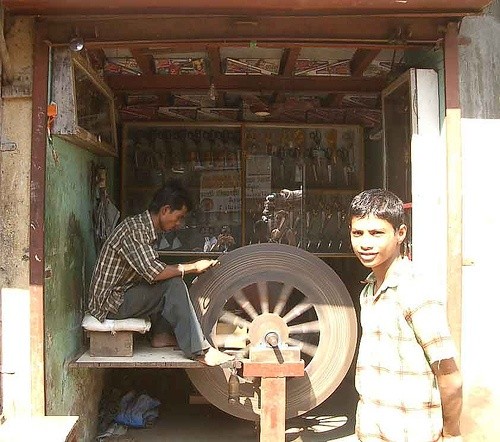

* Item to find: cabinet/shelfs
[120,123,365,263]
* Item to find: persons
[249,210,296,247]
[348,189,463,442]
[203,226,236,252]
[89,186,236,366]
